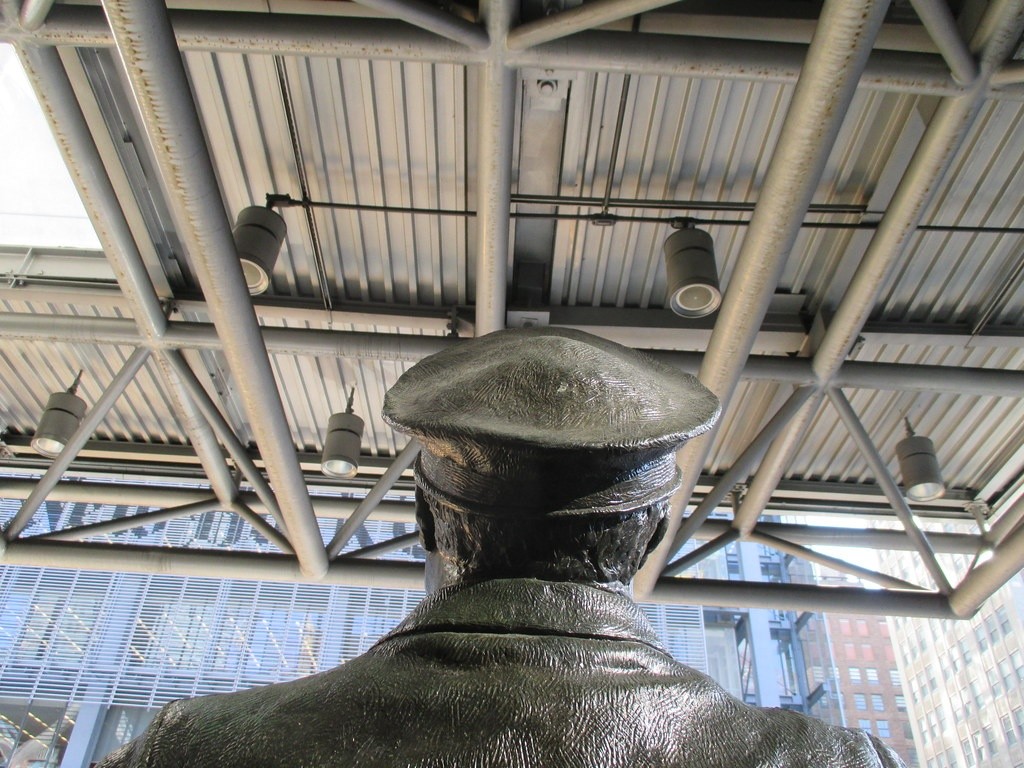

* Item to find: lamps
[895,416,947,502]
[230,193,291,297]
[321,386,364,478]
[31,368,87,458]
[664,221,722,318]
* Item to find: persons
[89,325,912,768]
